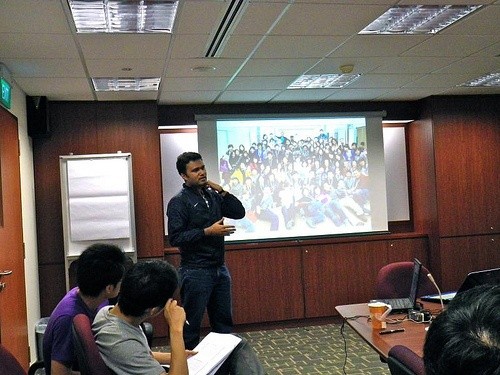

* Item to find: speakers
[27,96,50,137]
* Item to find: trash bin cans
[36,317,52,362]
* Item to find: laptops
[370,258,421,311]
[421,268,500,304]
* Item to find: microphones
[421,267,445,311]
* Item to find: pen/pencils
[170,299,190,324]
[378,328,405,334]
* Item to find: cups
[368,303,391,329]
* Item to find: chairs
[70,313,114,375]
[375,261,431,298]
[387,345,425,375]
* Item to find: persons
[91,259,268,375]
[220,129,370,232]
[423,284,500,375]
[166,152,245,350]
[43,244,126,375]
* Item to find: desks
[333,299,445,364]
